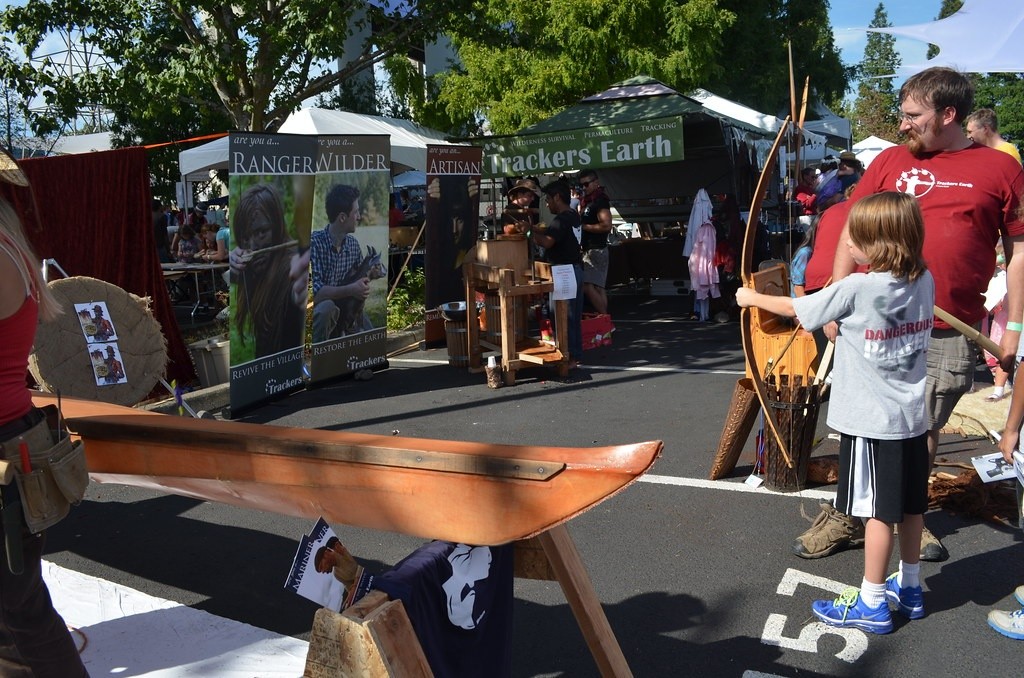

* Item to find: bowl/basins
[438,302,485,321]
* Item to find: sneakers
[918,525,947,560]
[791,498,866,558]
[885,571,925,619]
[1015,585,1024,606]
[987,609,1024,640]
[812,588,894,634]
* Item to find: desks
[161,261,228,326]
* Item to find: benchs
[746,263,831,385]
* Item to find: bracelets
[207,255,210,259]
[1005,322,1024,331]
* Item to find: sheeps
[329,246,388,338]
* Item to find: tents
[472,75,851,196]
[182,107,472,226]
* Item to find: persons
[229,184,310,359]
[791,66,1024,639]
[104,346,125,384]
[578,168,616,332]
[736,191,936,634]
[310,185,388,343]
[0,197,91,678]
[326,536,352,558]
[531,180,583,265]
[92,305,114,341]
[502,179,541,258]
[314,546,363,610]
[152,199,229,307]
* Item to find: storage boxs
[541,313,612,354]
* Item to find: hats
[0,145,28,187]
[837,153,855,160]
[508,179,543,197]
[196,202,208,215]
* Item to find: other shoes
[189,308,199,317]
[576,360,583,368]
[985,394,1004,402]
[610,323,616,333]
[197,306,210,315]
[567,361,576,370]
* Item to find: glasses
[579,179,594,188]
[899,105,945,125]
[810,175,817,179]
[545,197,553,203]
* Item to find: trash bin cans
[189,332,230,389]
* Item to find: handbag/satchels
[0,410,89,535]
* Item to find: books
[283,515,373,614]
